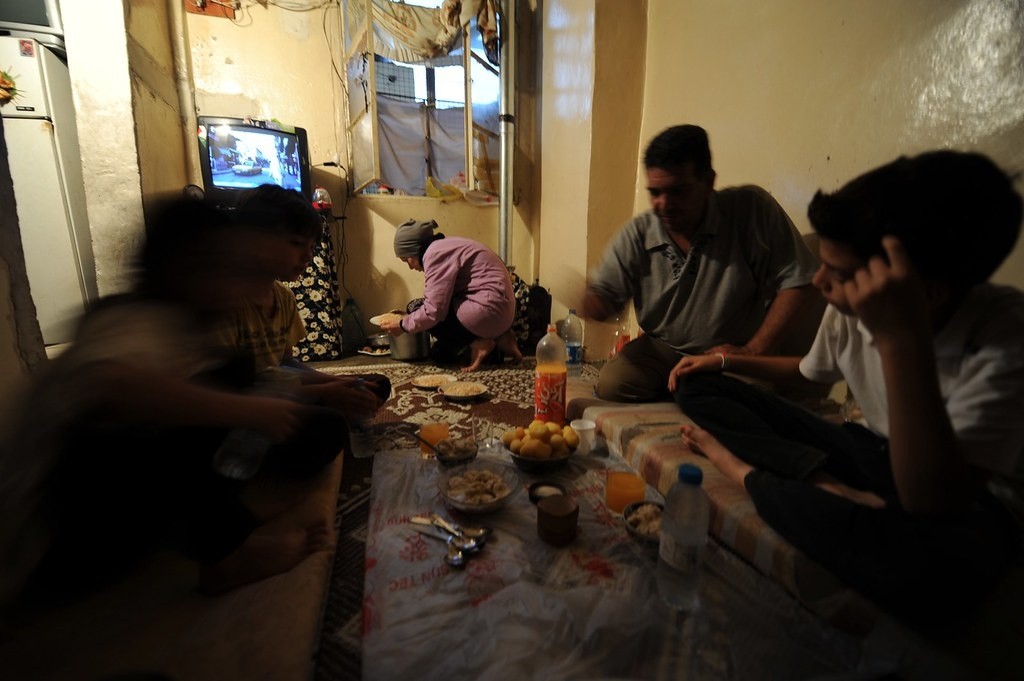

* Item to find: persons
[662,149,1023,657]
[551,119,813,407]
[377,217,523,374]
[0,182,333,676]
[200,181,395,478]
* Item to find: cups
[537,494,579,549]
[471,401,494,453]
[605,464,647,520]
[571,420,596,458]
[419,421,451,460]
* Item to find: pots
[389,327,430,362]
[366,334,389,346]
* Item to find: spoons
[409,511,493,568]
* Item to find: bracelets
[400,317,412,334]
[713,351,731,370]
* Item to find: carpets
[313,363,658,680]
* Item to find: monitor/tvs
[198,115,311,203]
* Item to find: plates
[411,374,458,388]
[358,349,391,355]
[437,380,487,398]
[369,315,383,325]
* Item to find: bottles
[657,464,710,610]
[559,310,583,386]
[343,378,376,459]
[534,324,566,429]
[608,307,631,364]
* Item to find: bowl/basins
[622,501,668,550]
[434,440,479,468]
[502,442,578,473]
[436,460,520,513]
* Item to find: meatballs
[504,420,580,460]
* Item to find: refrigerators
[0,36,101,346]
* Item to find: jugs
[312,188,333,209]
